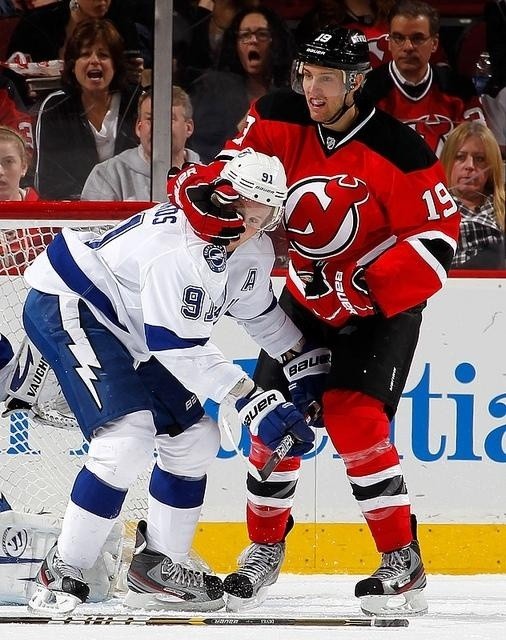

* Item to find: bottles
[471,51,492,93]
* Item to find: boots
[224,514,293,598]
[35,540,90,603]
[126,521,223,602]
[354,514,426,599]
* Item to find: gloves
[282,346,334,427]
[235,388,315,457]
[303,260,378,328]
[167,158,246,246]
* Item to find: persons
[167,26,461,599]
[0,0,506,268]
[22,146,332,605]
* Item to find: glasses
[236,29,274,44]
[386,31,435,47]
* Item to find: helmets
[221,147,290,233]
[290,27,373,98]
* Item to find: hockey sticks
[221,399,321,482]
